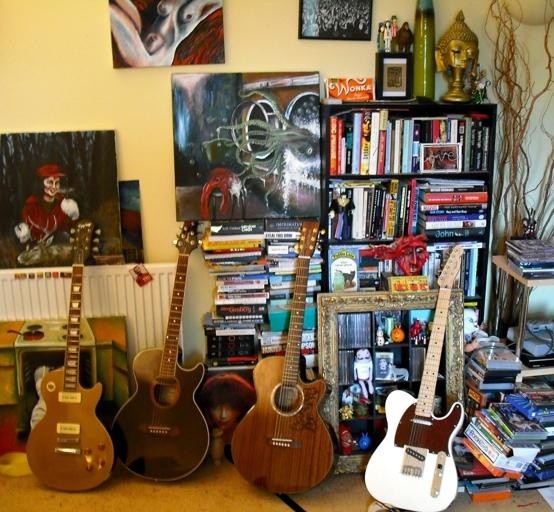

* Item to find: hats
[35,162,66,178]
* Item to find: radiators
[0,263,185,395]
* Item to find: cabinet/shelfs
[320,101,498,326]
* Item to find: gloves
[14,222,31,244]
[61,197,80,221]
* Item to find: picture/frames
[376,52,413,100]
[299,0,372,40]
[317,289,465,476]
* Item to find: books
[329,177,488,241]
[329,111,490,174]
[452,343,554,503]
[505,239,554,279]
[201,220,324,367]
[329,243,483,386]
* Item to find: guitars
[26,220,115,492]
[365,243,466,512]
[108,219,211,483]
[231,218,340,494]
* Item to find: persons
[435,10,480,103]
[383,21,391,54]
[202,373,257,466]
[338,349,374,455]
[131,266,151,286]
[317,1,370,39]
[376,22,386,53]
[13,165,79,251]
[390,15,398,54]
